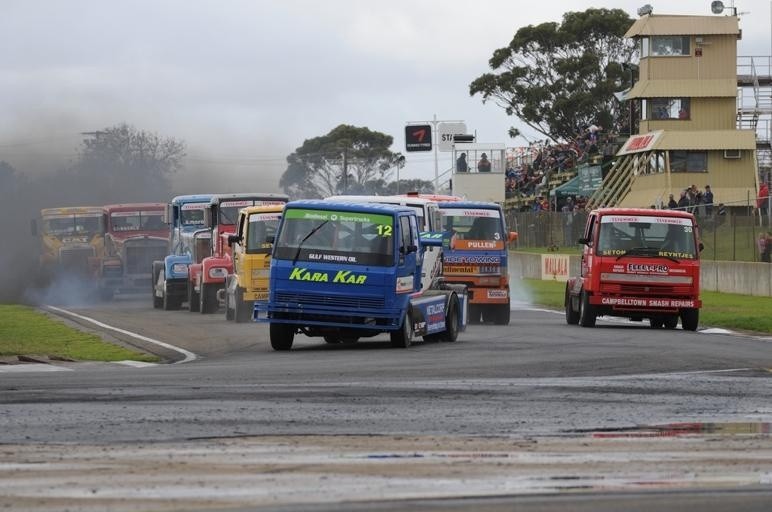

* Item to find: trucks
[187,193,289,314]
[434,201,518,325]
[151,195,215,311]
[322,195,469,332]
[28,206,104,292]
[252,199,461,351]
[384,193,462,203]
[225,204,288,324]
[564,207,704,330]
[89,203,171,302]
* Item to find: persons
[758,231,772,263]
[457,153,467,172]
[504,106,726,247]
[296,221,331,247]
[757,180,769,226]
[478,153,490,172]
